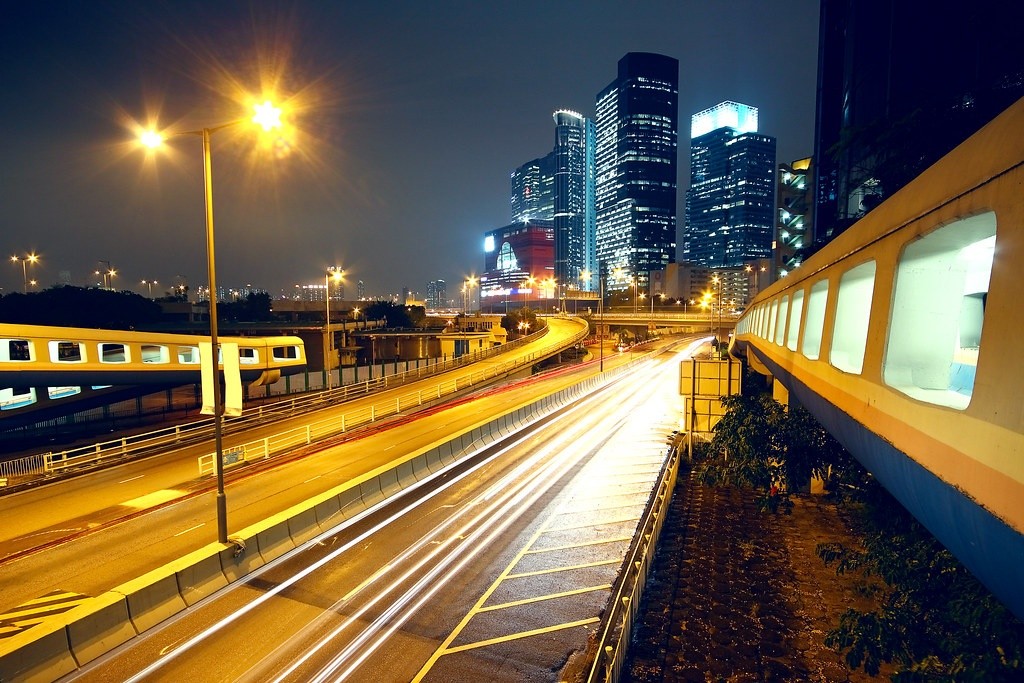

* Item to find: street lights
[525,278,534,335]
[519,279,555,323]
[142,105,277,543]
[630,276,644,317]
[640,294,665,352]
[746,266,765,296]
[706,294,713,336]
[464,280,475,354]
[714,278,721,361]
[9,255,186,297]
[581,271,622,372]
[677,301,694,319]
[358,291,456,315]
[326,272,342,400]
